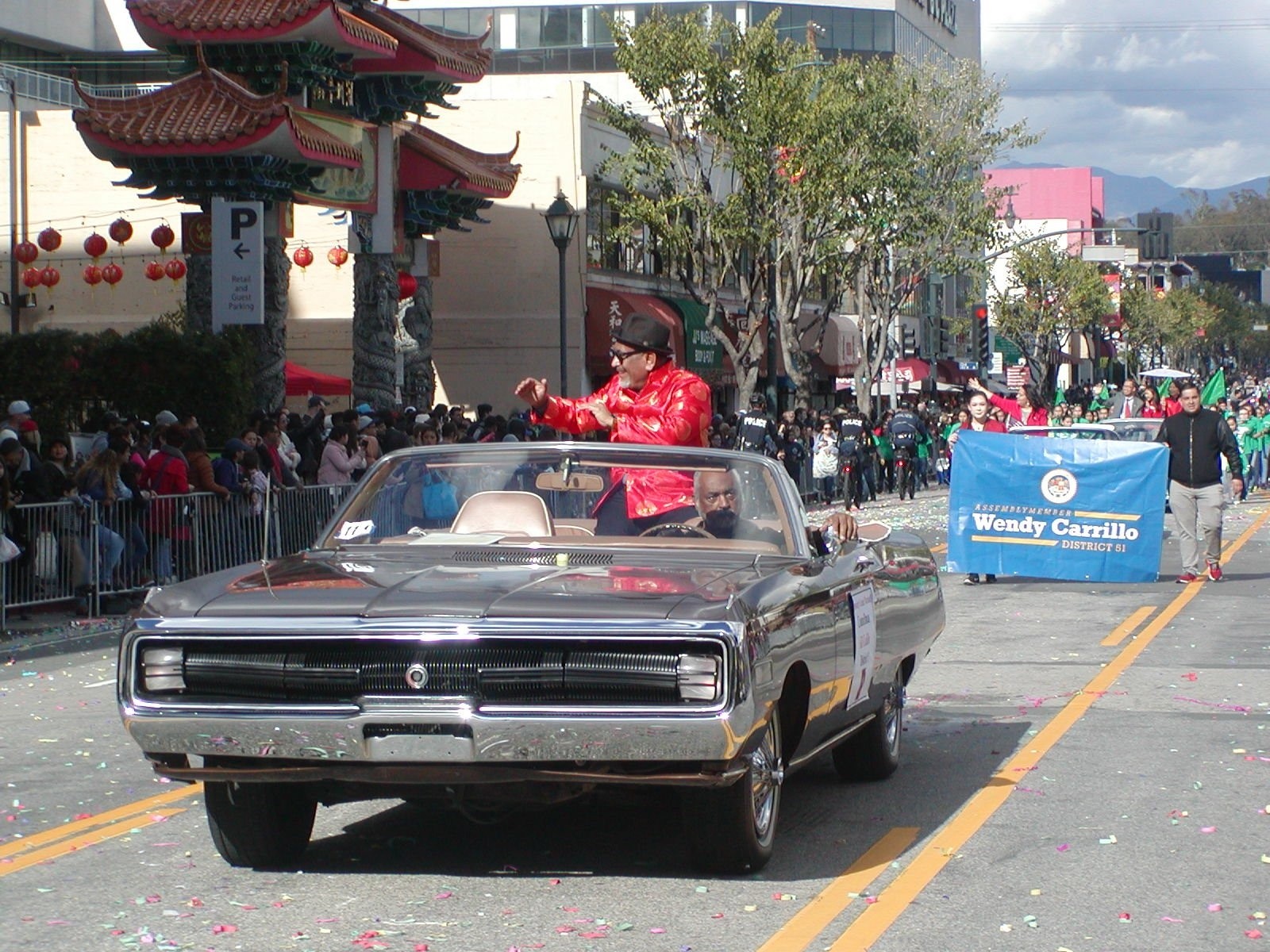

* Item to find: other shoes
[99,582,118,592]
[1175,571,1196,584]
[963,573,980,585]
[75,584,95,594]
[133,576,155,586]
[1205,559,1222,581]
[76,605,94,617]
[986,573,997,583]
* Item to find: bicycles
[892,445,915,500]
[833,437,880,510]
[728,448,784,520]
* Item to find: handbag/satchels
[0,534,21,563]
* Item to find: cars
[115,440,945,873]
[1002,417,1171,511]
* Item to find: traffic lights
[934,314,950,354]
[975,305,988,321]
[900,323,915,360]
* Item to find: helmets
[847,405,860,412]
[748,392,765,403]
[900,400,910,407]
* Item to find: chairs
[448,490,556,540]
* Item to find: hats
[96,409,121,431]
[155,410,178,426]
[225,438,250,457]
[307,396,330,408]
[7,400,30,415]
[610,312,675,357]
[357,416,373,432]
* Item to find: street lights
[541,191,582,442]
[765,61,836,452]
[940,192,1020,359]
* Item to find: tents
[285,360,351,409]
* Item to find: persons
[968,377,1048,436]
[1202,374,1270,504]
[682,468,858,548]
[710,393,969,513]
[515,313,712,535]
[1046,377,1182,440]
[946,391,1009,585]
[0,400,303,620]
[1155,383,1244,583]
[276,396,609,552]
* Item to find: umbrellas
[1139,369,1192,377]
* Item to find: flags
[1090,382,1109,411]
[1158,377,1173,397]
[1201,370,1226,405]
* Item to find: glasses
[608,346,647,365]
[280,418,290,425]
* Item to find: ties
[1125,399,1130,418]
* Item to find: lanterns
[15,218,187,302]
[396,269,417,302]
[293,246,314,272]
[328,246,348,269]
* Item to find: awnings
[584,285,1041,394]
[1094,339,1117,358]
[1049,348,1081,364]
